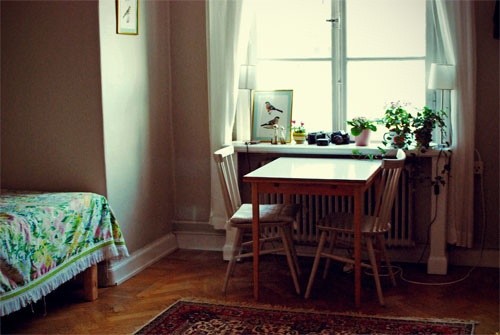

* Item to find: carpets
[131,296,477,335]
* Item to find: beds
[0,189,129,322]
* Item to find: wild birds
[260,116,280,129]
[265,102,283,115]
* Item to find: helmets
[264,97,276,107]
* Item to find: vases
[292,132,306,145]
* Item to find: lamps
[427,61,457,147]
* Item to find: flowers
[293,120,306,131]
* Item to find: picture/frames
[115,0,140,35]
[249,88,292,144]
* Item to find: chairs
[213,145,302,295]
[305,148,406,307]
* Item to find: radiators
[258,159,417,249]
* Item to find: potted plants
[347,103,446,147]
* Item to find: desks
[242,156,385,307]
